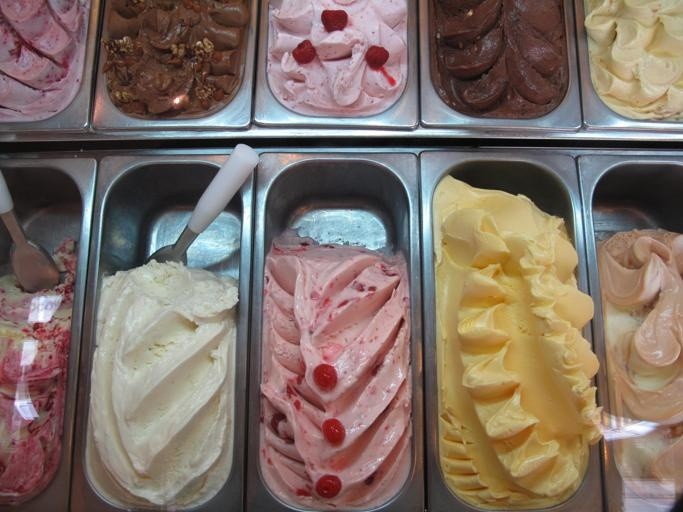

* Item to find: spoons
[1,170,60,293]
[146,143,260,267]
